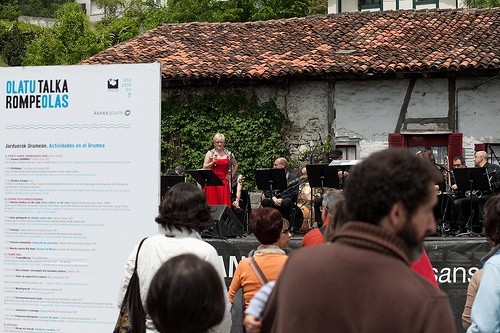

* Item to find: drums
[299,183,317,201]
[292,203,315,233]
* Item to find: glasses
[453,163,463,167]
[281,227,291,234]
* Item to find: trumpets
[443,157,454,195]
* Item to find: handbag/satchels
[113,237,148,333]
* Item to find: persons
[227,208,288,333]
[302,190,344,247]
[202,133,237,208]
[260,148,456,333]
[244,280,276,333]
[147,253,225,333]
[412,247,439,290]
[461,269,481,329]
[464,195,500,333]
[264,157,299,238]
[277,219,293,255]
[328,200,348,233]
[118,182,233,333]
[328,149,349,186]
[416,151,500,237]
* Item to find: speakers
[200,205,244,237]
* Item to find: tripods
[452,167,492,238]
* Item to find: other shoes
[469,231,480,236]
[455,228,467,235]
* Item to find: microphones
[214,153,218,167]
[450,171,453,174]
[319,134,323,145]
